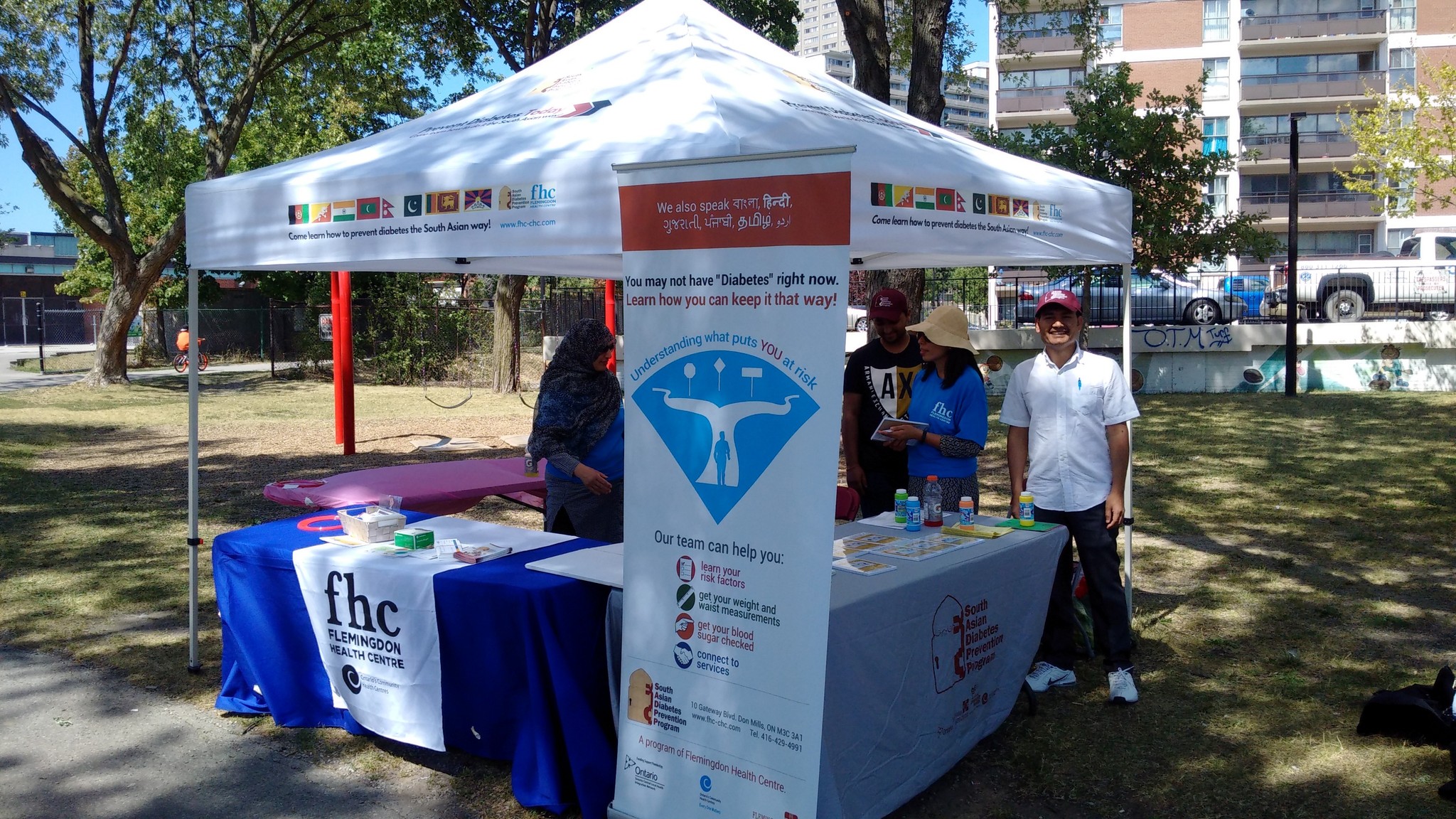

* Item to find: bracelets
[919,430,927,443]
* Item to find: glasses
[599,342,615,354]
[916,332,933,344]
[873,317,900,327]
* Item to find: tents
[181,0,1136,675]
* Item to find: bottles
[894,489,908,523]
[959,497,974,531]
[525,445,539,477]
[1019,492,1034,527]
[906,496,921,531]
[923,475,943,527]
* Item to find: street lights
[1283,110,1308,397]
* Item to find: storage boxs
[393,528,434,550]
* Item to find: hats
[1036,289,1083,317]
[905,306,980,355]
[869,288,907,321]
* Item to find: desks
[212,457,1068,818]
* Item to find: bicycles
[173,338,209,373]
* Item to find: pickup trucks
[1259,232,1456,323]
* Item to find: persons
[841,288,925,521]
[177,326,205,366]
[998,288,1140,706]
[527,317,623,543]
[878,306,988,515]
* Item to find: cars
[1216,275,1276,317]
[846,305,868,332]
[1014,263,1248,326]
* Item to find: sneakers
[1022,659,1077,691]
[1106,665,1138,703]
